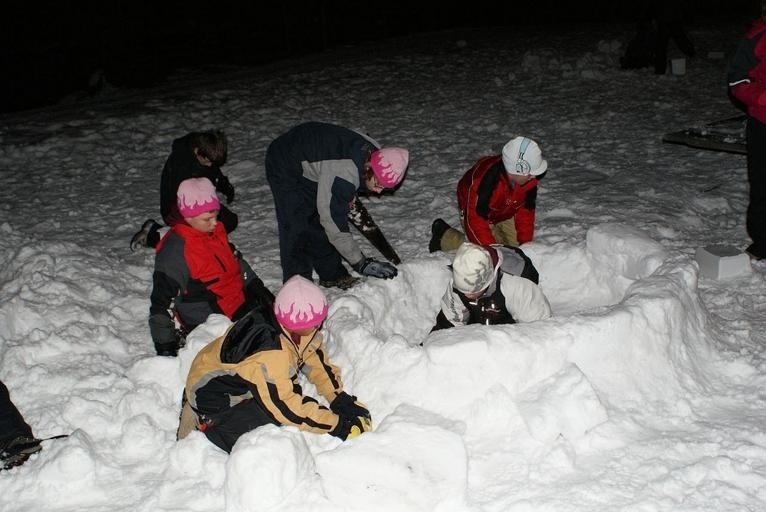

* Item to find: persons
[176,274,372,455]
[420,238,553,348]
[0,381,43,471]
[128,126,239,250]
[726,14,766,262]
[148,177,276,358]
[263,120,411,291]
[424,135,551,253]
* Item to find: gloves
[327,416,373,441]
[152,340,179,356]
[352,258,399,280]
[330,391,371,420]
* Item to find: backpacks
[487,242,538,285]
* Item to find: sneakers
[131,218,155,251]
[319,273,362,290]
[0,434,42,471]
[429,217,450,254]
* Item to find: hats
[370,144,410,190]
[450,241,502,294]
[502,136,548,176]
[274,273,329,331]
[176,177,221,218]
